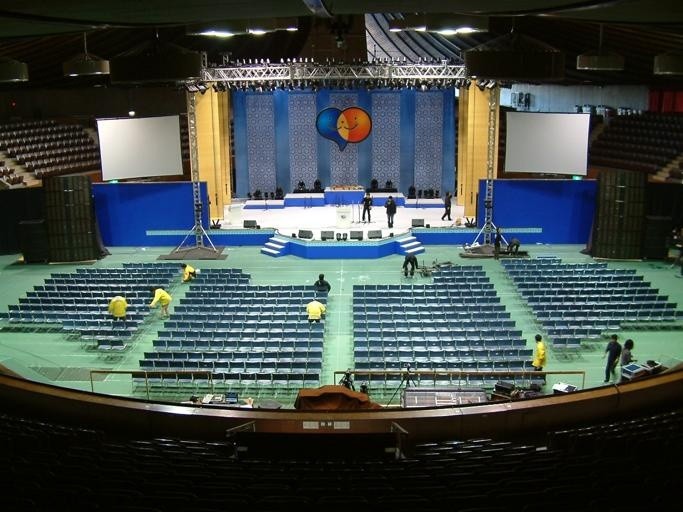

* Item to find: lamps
[2,58,28,84]
[63,32,110,79]
[575,23,681,78]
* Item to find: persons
[531,334,547,386]
[601,334,622,383]
[507,238,521,257]
[440,191,453,222]
[384,196,397,229]
[305,299,326,323]
[148,287,172,320]
[108,294,128,322]
[314,273,331,293]
[402,254,419,279]
[180,263,197,282]
[493,227,503,261]
[620,338,639,382]
[360,193,373,223]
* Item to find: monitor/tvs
[224,392,238,404]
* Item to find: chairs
[589,113,681,174]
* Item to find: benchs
[0,112,188,189]
